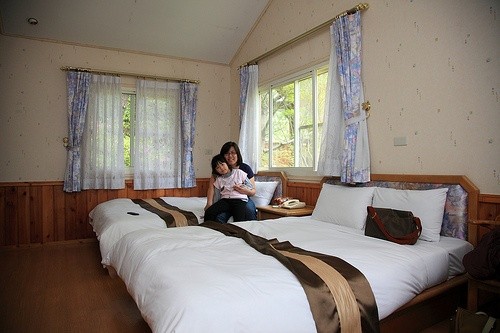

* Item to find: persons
[204,154,255,222]
[204,141,257,222]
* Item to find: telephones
[282,199,306,209]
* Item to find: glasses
[224,152,237,156]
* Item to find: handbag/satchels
[365,206,422,245]
